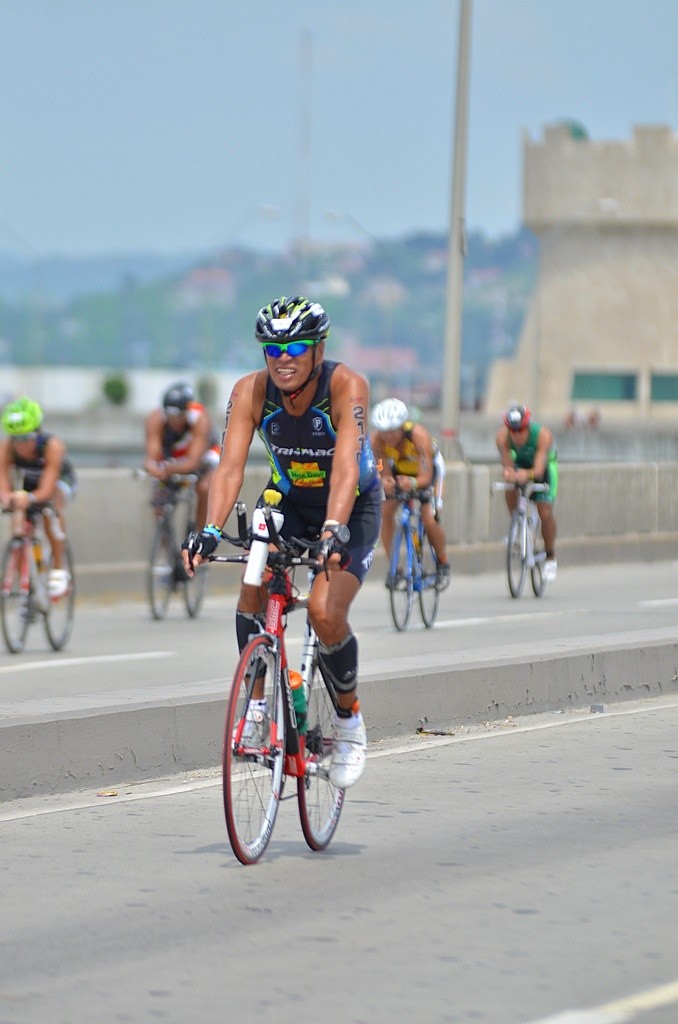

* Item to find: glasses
[259,338,320,358]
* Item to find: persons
[143,382,223,587]
[368,398,452,592]
[181,296,386,789]
[495,405,559,581]
[0,396,76,602]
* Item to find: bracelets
[203,523,223,542]
[412,478,417,490]
[531,471,534,480]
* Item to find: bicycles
[181,501,354,861]
[381,484,454,630]
[145,464,217,621]
[1,500,79,656]
[489,479,560,598]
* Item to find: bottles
[412,527,420,557]
[33,537,44,575]
[288,670,310,734]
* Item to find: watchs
[324,524,350,544]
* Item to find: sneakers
[385,568,404,588]
[230,709,272,750]
[543,555,558,581]
[327,716,366,788]
[49,566,71,599]
[433,560,450,591]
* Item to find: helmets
[372,396,409,433]
[503,404,532,432]
[2,395,42,436]
[163,383,194,411]
[257,295,330,343]
